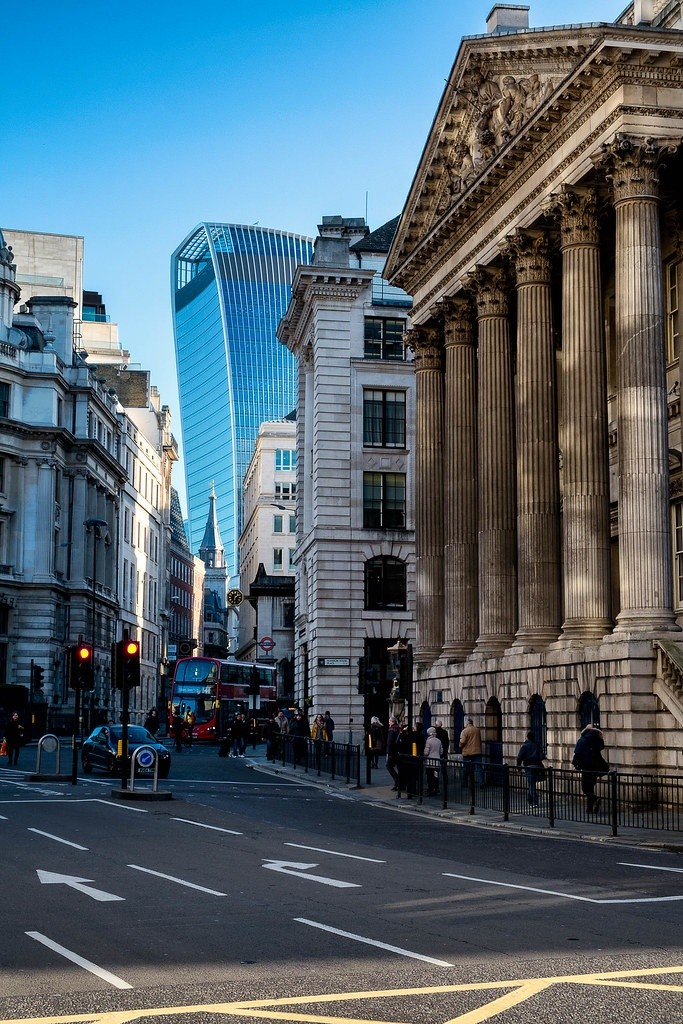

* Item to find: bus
[168,656,276,744]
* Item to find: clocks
[227,589,244,606]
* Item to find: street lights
[385,640,407,730]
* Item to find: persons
[517,732,539,809]
[2,711,25,765]
[173,711,184,753]
[434,719,449,759]
[371,716,384,769]
[229,714,249,759]
[261,710,309,760]
[386,717,427,799]
[424,727,443,797]
[184,720,194,748]
[144,710,160,735]
[312,711,335,742]
[449,72,540,180]
[459,719,485,792]
[572,724,605,814]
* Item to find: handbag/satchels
[537,762,547,781]
[597,756,610,777]
[0,738,7,756]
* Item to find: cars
[80,724,172,778]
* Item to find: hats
[325,711,330,715]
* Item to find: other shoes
[529,803,539,808]
[231,754,237,758]
[375,762,378,769]
[238,755,245,757]
[593,798,601,814]
[371,762,375,768]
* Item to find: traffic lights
[33,665,44,690]
[116,638,140,688]
[76,646,93,690]
